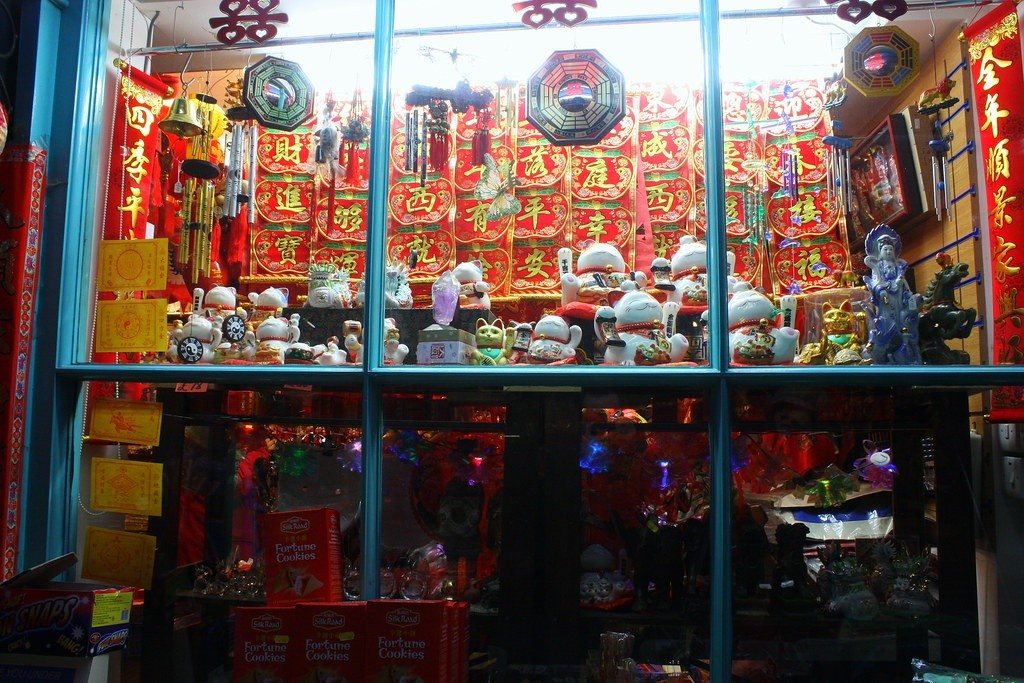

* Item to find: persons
[862,235,922,365]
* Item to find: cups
[599,633,634,683]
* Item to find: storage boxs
[0,552,134,683]
[417,329,478,365]
[267,507,344,607]
[233,599,470,683]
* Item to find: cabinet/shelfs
[140,383,981,683]
